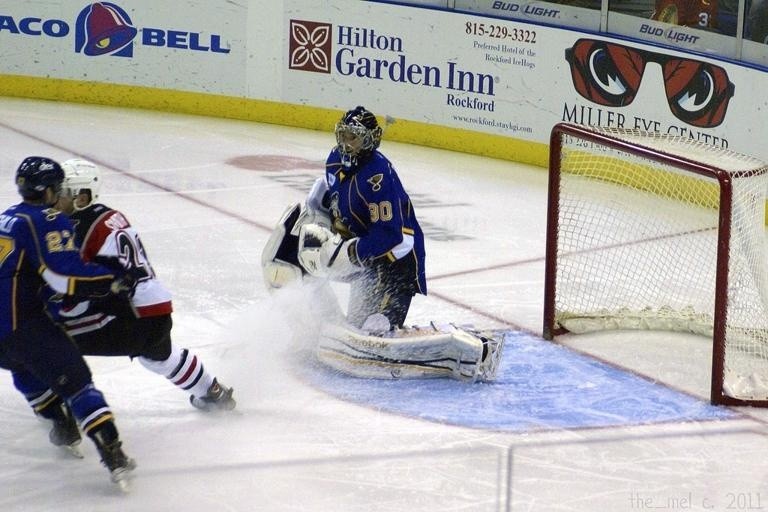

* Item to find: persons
[0,156,141,488]
[39,156,240,412]
[259,106,506,386]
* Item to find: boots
[189,377,236,410]
[48,402,81,445]
[87,421,138,470]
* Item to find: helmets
[60,159,101,199]
[15,156,64,202]
[334,106,382,152]
